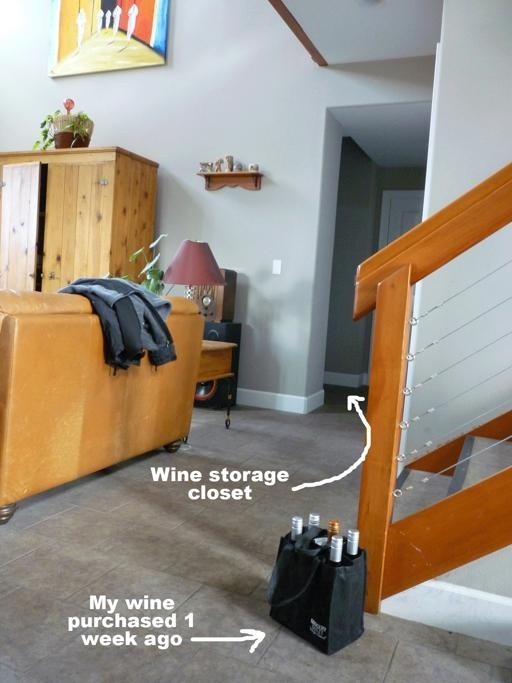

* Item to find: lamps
[161,239,228,300]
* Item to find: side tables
[197,340,239,430]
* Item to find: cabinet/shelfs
[1,146,159,293]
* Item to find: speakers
[193,269,236,323]
[193,322,241,410]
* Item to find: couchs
[1,289,205,527]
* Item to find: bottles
[289,510,362,565]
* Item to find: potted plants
[31,109,95,151]
